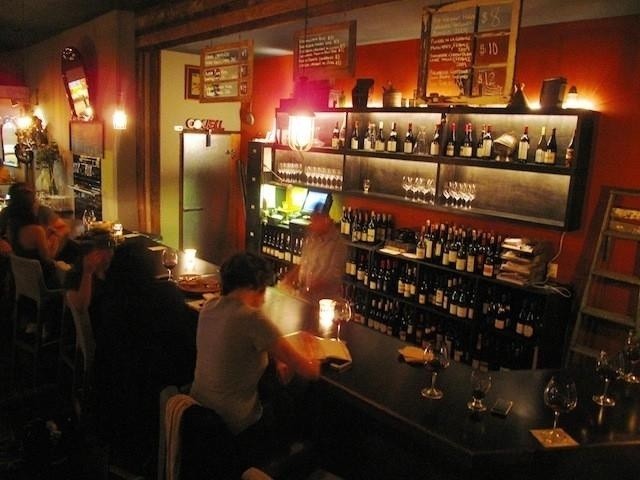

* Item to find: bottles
[505,82,532,112]
[330,118,577,168]
[254,195,549,371]
[337,90,347,108]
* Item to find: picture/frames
[292,19,359,82]
[198,36,255,102]
[414,1,525,105]
[184,63,205,101]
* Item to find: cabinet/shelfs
[260,220,573,372]
[497,235,553,287]
[274,108,598,231]
[245,141,274,269]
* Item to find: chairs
[241,467,343,480]
[156,383,310,480]
[58,287,149,480]
[9,252,68,385]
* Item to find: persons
[280,204,347,306]
[64,229,117,428]
[1,182,73,259]
[183,249,327,480]
[89,236,200,456]
[7,186,79,339]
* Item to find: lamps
[569,85,577,96]
[287,2,315,151]
[109,1,128,129]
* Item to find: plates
[176,279,222,293]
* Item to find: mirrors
[2,116,20,169]
[60,46,91,122]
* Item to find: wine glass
[592,345,626,408]
[401,174,479,210]
[83,210,95,232]
[161,248,179,284]
[362,177,371,193]
[463,367,494,414]
[420,336,451,400]
[276,162,343,191]
[620,329,640,385]
[541,371,580,444]
